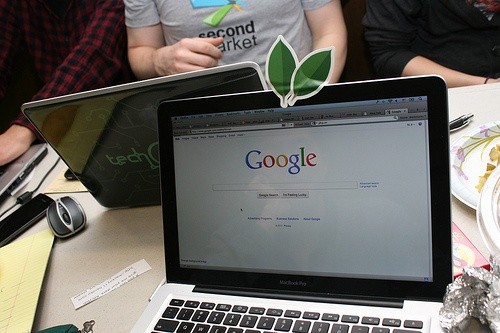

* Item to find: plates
[450,120,500,210]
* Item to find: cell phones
[0,193,54,248]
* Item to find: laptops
[129,77,454,333]
[22,60,268,210]
[0,143,48,206]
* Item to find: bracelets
[484,78,489,84]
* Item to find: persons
[361,0,500,89]
[0,0,126,166]
[121,0,348,91]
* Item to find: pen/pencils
[450,113,474,133]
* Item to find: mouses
[46,196,87,238]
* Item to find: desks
[0,83,491,333]
